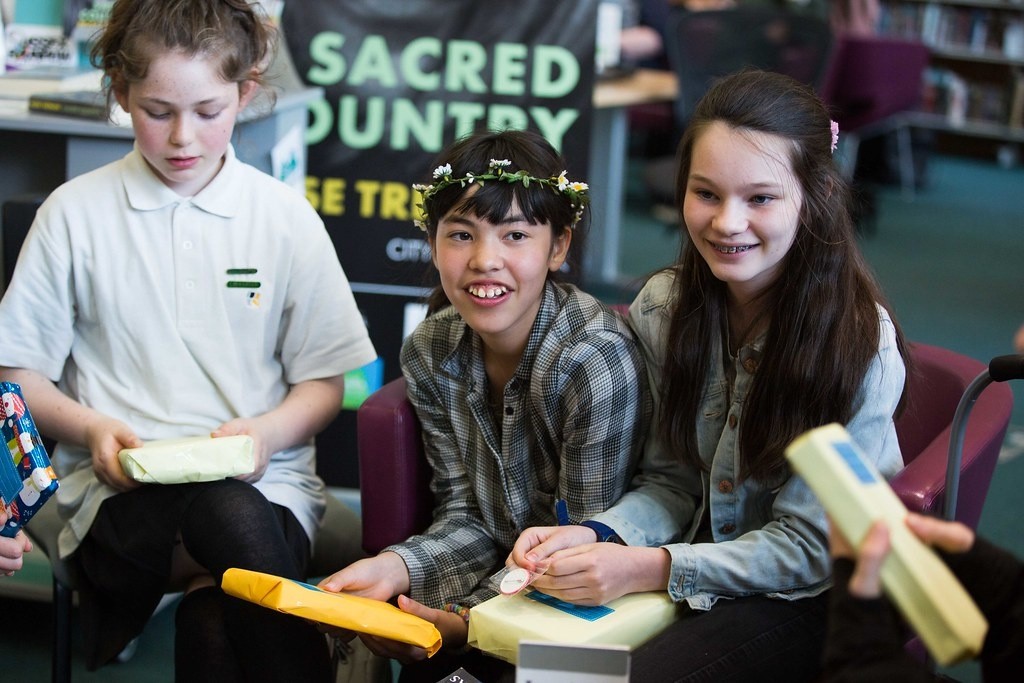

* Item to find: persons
[505,74,906,683]
[823,506,1024,683]
[317,132,651,683]
[1,0,377,683]
[826,0,929,201]
[622,0,735,55]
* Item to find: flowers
[828,119,839,153]
[413,158,588,232]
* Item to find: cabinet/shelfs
[879,0,1024,170]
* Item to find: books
[880,0,1024,123]
[29,90,130,121]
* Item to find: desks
[0,85,324,282]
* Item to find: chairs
[670,8,930,225]
[356,338,1013,682]
